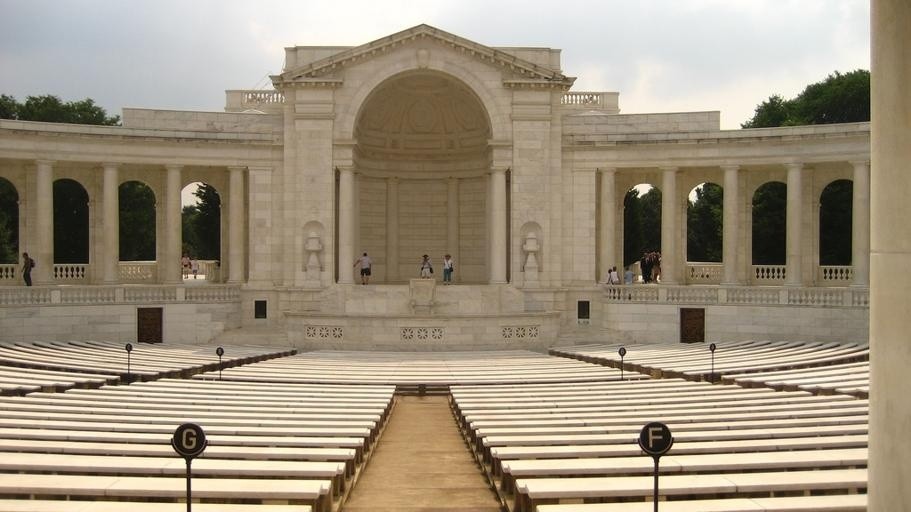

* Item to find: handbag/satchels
[429,267,433,274]
[449,268,453,273]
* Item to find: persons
[181,253,191,279]
[607,269,613,293]
[641,250,661,284]
[20,253,32,286]
[624,267,635,285]
[421,255,432,278]
[443,255,453,285]
[192,257,199,279]
[353,252,372,285]
[606,266,621,299]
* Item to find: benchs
[548,338,869,400]
[192,348,651,396]
[0,377,397,511]
[0,339,297,398]
[448,378,869,510]
